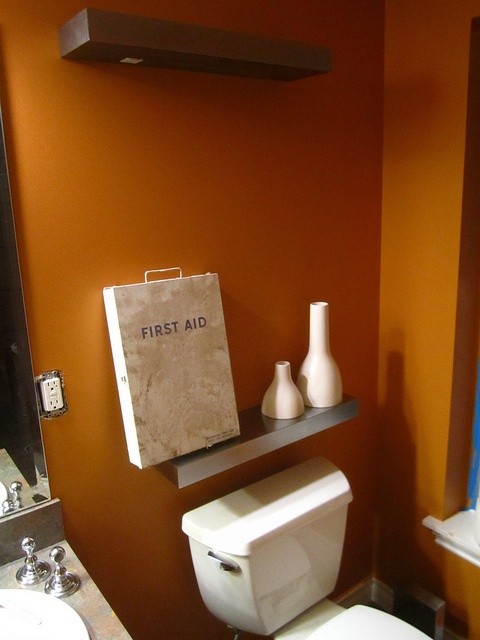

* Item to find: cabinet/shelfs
[154,392,362,490]
[58,10,333,84]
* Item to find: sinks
[1,482,9,504]
[2,590,89,640]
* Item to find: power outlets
[39,378,65,411]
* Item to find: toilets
[180,454,432,638]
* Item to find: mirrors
[0,115,51,518]
